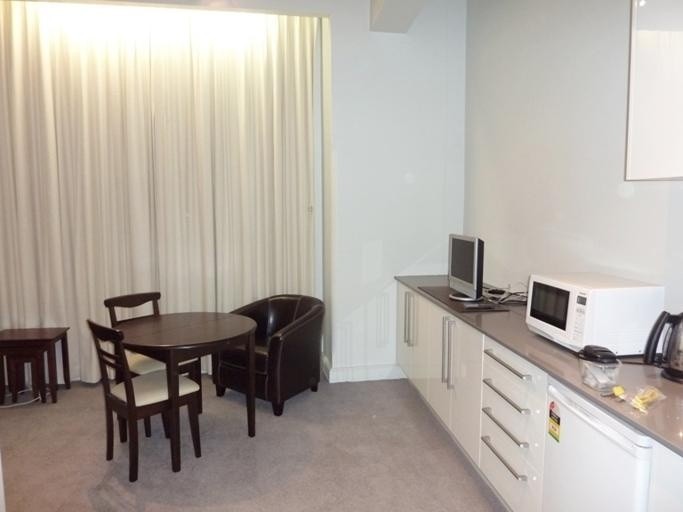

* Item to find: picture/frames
[622,0,683,182]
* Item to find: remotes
[465,302,494,310]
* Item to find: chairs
[214,291,326,418]
[84,316,204,483]
[103,292,204,419]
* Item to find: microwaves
[524,270,669,357]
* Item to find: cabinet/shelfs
[394,279,425,404]
[425,299,483,472]
[479,333,548,512]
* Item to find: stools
[0,345,51,406]
[0,324,72,410]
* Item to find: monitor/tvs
[449,233,484,301]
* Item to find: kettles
[643,311,683,383]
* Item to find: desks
[102,311,259,475]
